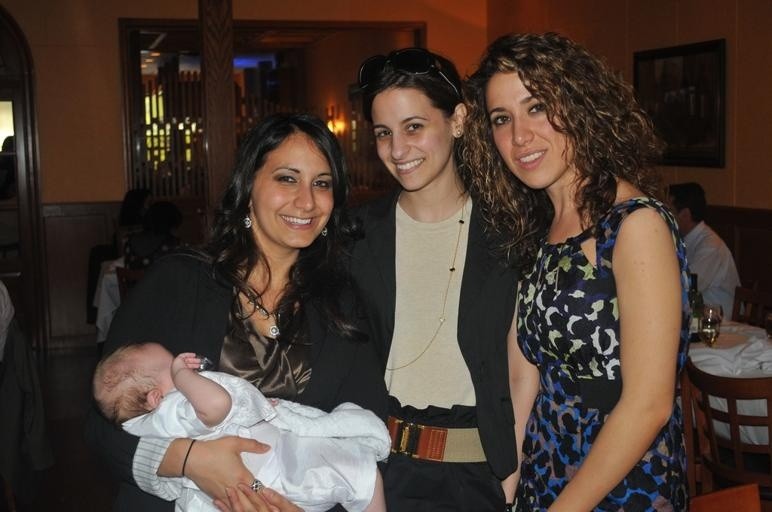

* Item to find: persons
[455,32,693,510]
[93,114,389,512]
[346,49,519,512]
[665,182,748,324]
[94,343,392,512]
[124,203,183,289]
[104,187,155,261]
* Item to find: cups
[698,305,723,348]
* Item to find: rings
[249,479,263,491]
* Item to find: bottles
[689,273,703,317]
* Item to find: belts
[387,416,487,463]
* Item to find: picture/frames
[633,38,726,168]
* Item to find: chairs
[115,267,146,306]
[682,285,771,511]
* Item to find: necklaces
[385,186,464,372]
[244,294,280,337]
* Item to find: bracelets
[182,440,197,476]
[174,368,191,382]
